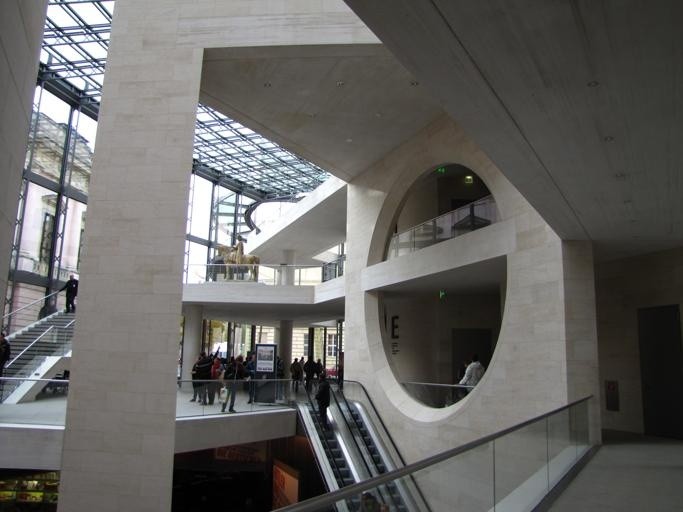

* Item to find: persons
[229,235,243,264]
[313,372,330,430]
[0,331,11,376]
[188,351,324,412]
[458,352,485,394]
[58,274,78,312]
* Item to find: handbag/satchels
[219,387,228,404]
